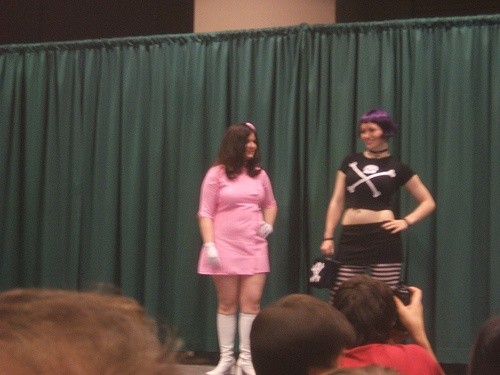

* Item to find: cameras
[394,288,410,307]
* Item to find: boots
[206,313,257,375]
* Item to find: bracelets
[322,237,335,241]
[402,218,412,230]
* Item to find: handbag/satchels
[307,255,343,288]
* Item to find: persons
[197,123,278,375]
[0,287,183,375]
[250,275,500,375]
[320,108,437,307]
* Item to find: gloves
[203,242,221,266]
[258,221,273,239]
